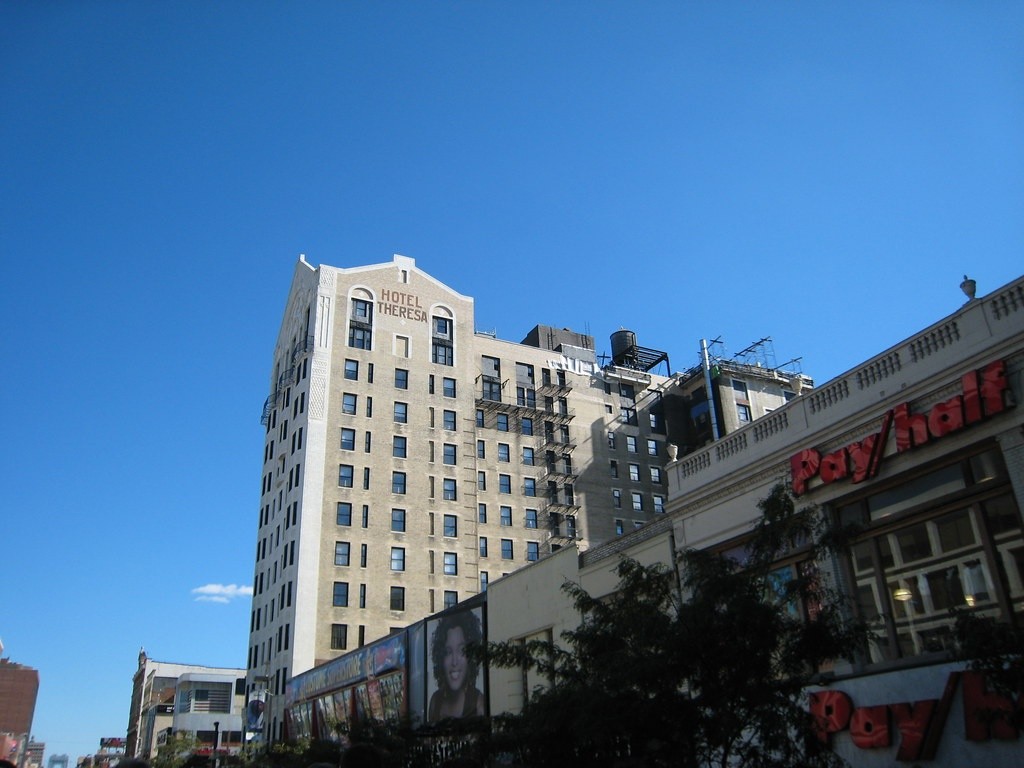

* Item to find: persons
[428,609,483,728]
[943,558,990,607]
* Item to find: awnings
[191,749,230,756]
